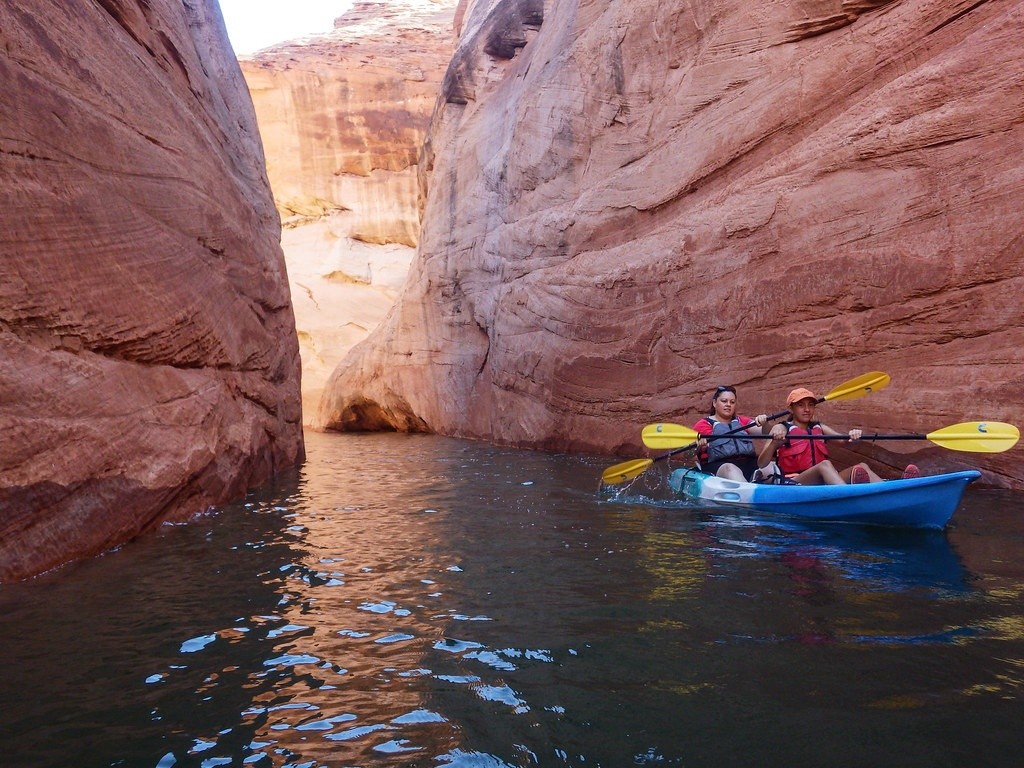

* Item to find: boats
[667,468,983,532]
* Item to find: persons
[684,387,781,483]
[758,388,919,485]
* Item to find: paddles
[601,370,892,487]
[640,420,1021,453]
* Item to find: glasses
[715,386,737,398]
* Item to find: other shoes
[749,470,763,483]
[850,465,870,484]
[900,464,920,479]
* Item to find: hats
[785,388,818,413]
